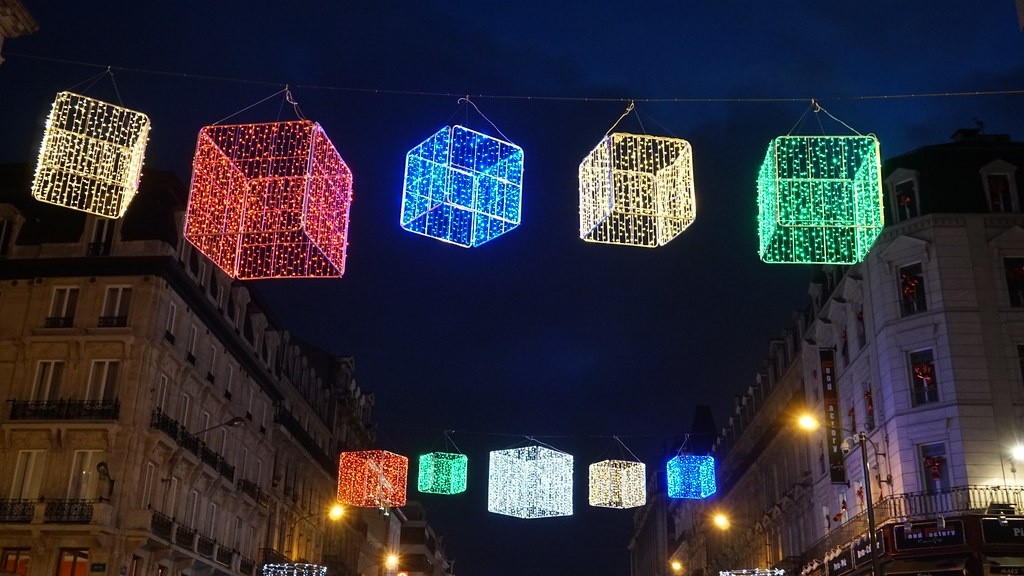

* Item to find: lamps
[289,503,343,542]
[712,510,776,567]
[180,417,245,444]
[796,409,892,487]
[670,557,702,576]
[94,460,117,495]
[358,551,401,574]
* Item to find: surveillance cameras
[841,443,850,454]
